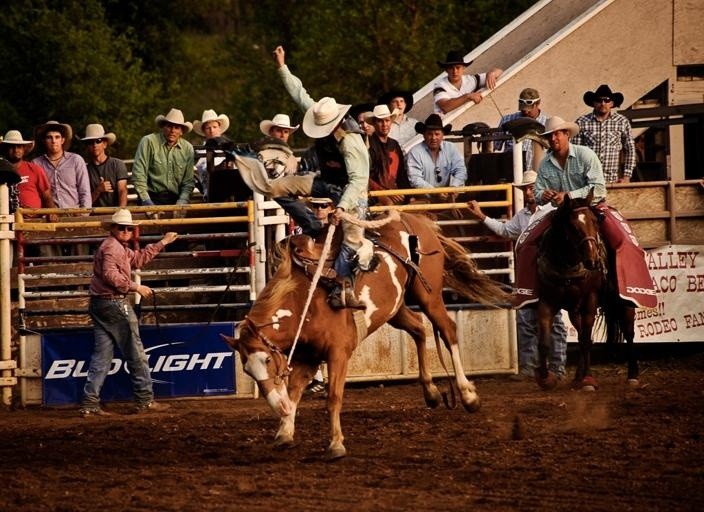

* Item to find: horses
[531,185,641,391]
[219,209,518,461]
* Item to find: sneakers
[301,377,330,401]
[509,371,536,382]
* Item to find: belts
[98,294,126,299]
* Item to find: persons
[75,124,129,208]
[80,209,178,417]
[404,113,467,238]
[131,108,195,219]
[367,104,411,205]
[259,114,300,142]
[433,53,503,116]
[377,88,421,146]
[531,117,608,208]
[351,103,376,137]
[269,45,371,312]
[291,196,335,396]
[2,130,60,257]
[571,85,635,183]
[468,170,568,380]
[191,109,238,203]
[492,88,551,171]
[30,121,93,257]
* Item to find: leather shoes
[80,407,112,417]
[328,292,367,310]
[136,401,171,412]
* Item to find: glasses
[118,225,134,231]
[518,98,541,106]
[434,166,442,183]
[595,97,611,103]
[310,202,331,208]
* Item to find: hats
[101,208,140,231]
[259,114,300,138]
[511,170,538,189]
[155,108,193,136]
[415,113,452,134]
[583,85,624,108]
[349,89,413,125]
[301,96,352,139]
[436,51,474,69]
[305,197,333,209]
[193,109,230,137]
[0,120,117,154]
[536,115,580,138]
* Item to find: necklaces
[50,156,62,161]
